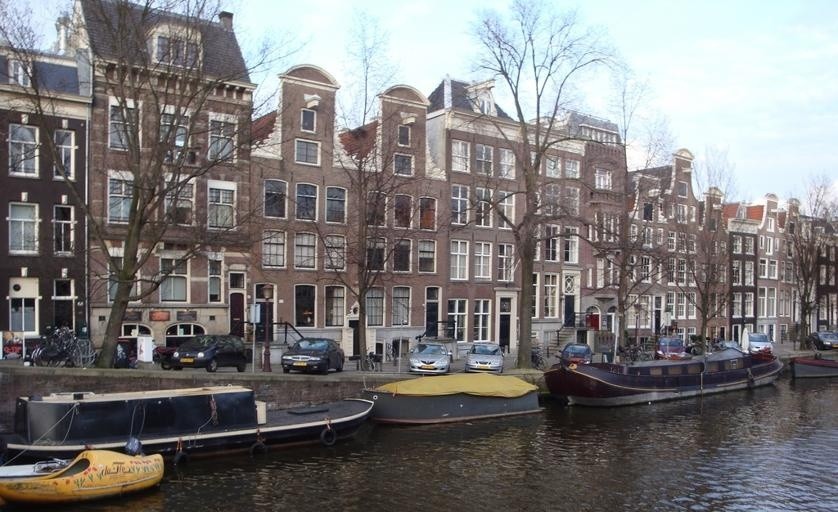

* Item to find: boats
[792,352,838,378]
[543,354,784,408]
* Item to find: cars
[748,331,774,355]
[658,336,684,360]
[280,337,346,375]
[407,343,450,374]
[174,335,248,372]
[558,342,595,364]
[807,331,838,351]
[465,341,504,375]
